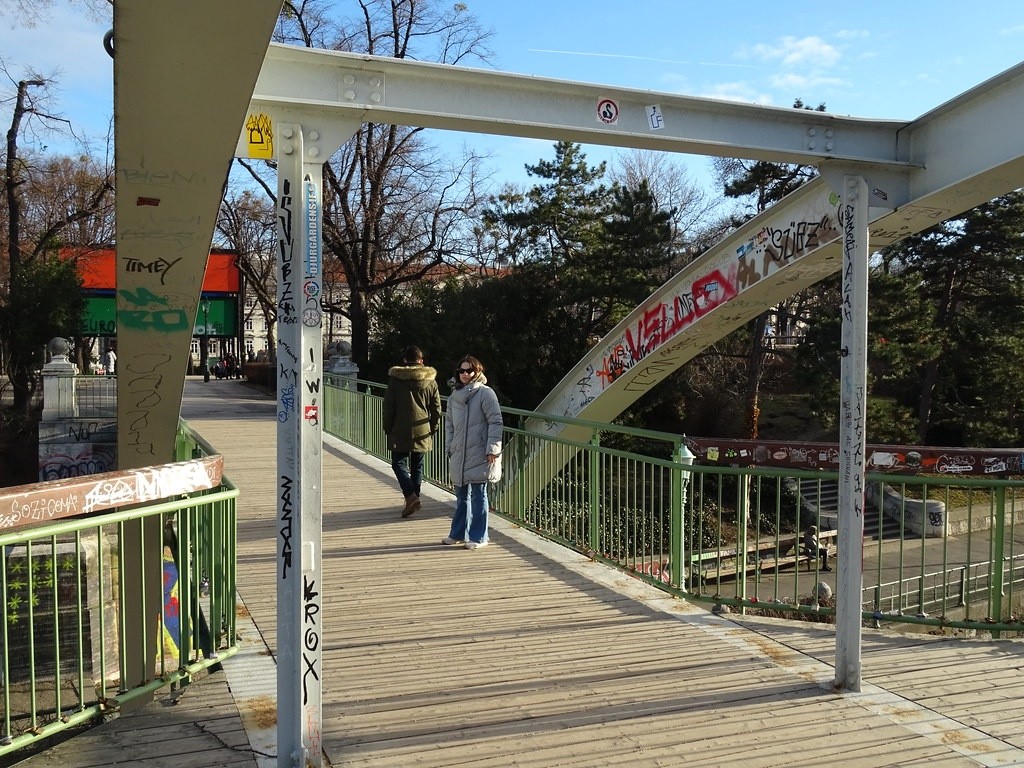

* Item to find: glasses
[456,368,475,374]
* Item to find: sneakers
[465,539,488,550]
[401,493,421,517]
[416,497,422,511]
[442,537,470,545]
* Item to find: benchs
[691,529,837,594]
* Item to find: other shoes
[822,565,833,571]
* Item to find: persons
[381,345,443,519]
[804,524,832,571]
[104,346,118,380]
[223,346,255,380]
[441,355,503,550]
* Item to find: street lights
[200,296,211,383]
